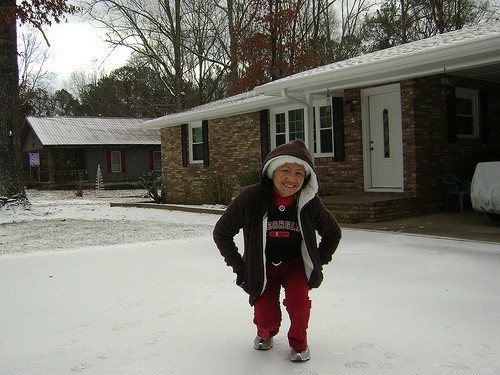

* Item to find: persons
[211,139,339,362]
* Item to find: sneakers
[289,346,311,362]
[254,335,273,351]
